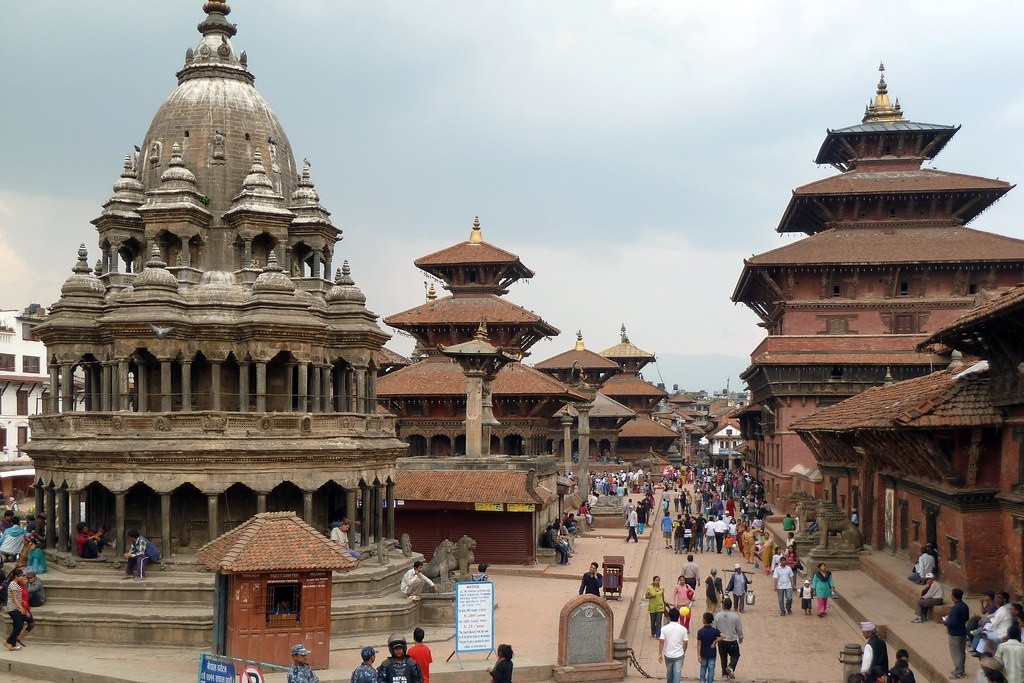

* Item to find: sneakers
[721,674,729,680]
[726,667,735,679]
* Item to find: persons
[400,561,441,600]
[472,563,492,582]
[658,598,744,682]
[847,541,1024,683]
[288,627,433,683]
[544,449,837,636]
[486,644,515,683]
[1,492,160,650]
[329,514,361,572]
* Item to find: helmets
[388,633,407,658]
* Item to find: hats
[804,580,810,583]
[291,644,311,655]
[924,572,936,579]
[26,571,36,578]
[735,564,740,569]
[981,590,995,597]
[361,646,379,659]
[860,622,875,630]
[478,562,489,572]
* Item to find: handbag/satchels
[832,591,838,599]
[686,584,693,600]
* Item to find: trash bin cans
[600,555,625,600]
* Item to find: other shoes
[739,609,744,613]
[413,596,422,601]
[948,671,965,679]
[406,595,416,598]
[788,610,792,614]
[911,617,927,623]
[781,611,785,616]
[4,642,15,650]
[17,638,27,646]
